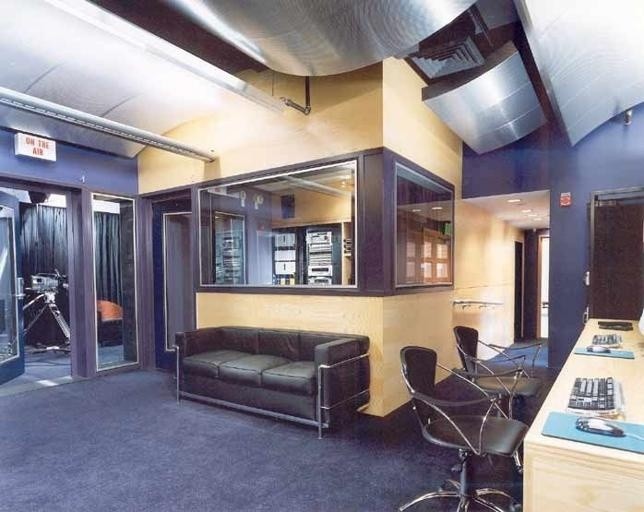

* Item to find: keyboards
[592,334,622,348]
[568,377,622,414]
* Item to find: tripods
[9,291,70,354]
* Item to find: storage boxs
[440,225,452,234]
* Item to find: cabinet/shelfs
[402,228,451,282]
[270,223,352,286]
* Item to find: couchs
[172,325,370,439]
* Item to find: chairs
[454,326,543,418]
[97,299,121,345]
[394,347,529,511]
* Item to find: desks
[524,318,644,511]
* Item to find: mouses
[586,345,610,353]
[576,416,624,437]
